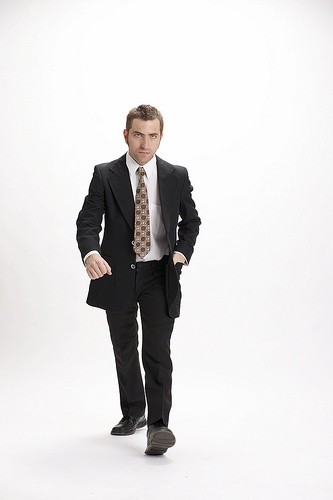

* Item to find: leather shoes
[110,412,147,435]
[145,425,175,455]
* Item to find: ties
[133,167,151,257]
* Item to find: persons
[76,105,202,457]
